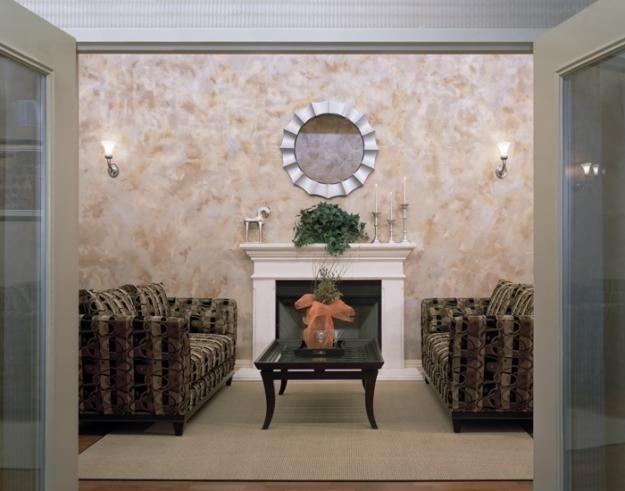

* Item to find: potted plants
[295,254,356,349]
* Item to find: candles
[374,176,406,219]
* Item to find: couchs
[420,280,537,433]
[80,282,238,436]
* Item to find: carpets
[79,381,534,481]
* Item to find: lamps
[102,140,118,178]
[496,142,510,178]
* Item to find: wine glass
[312,330,329,355]
